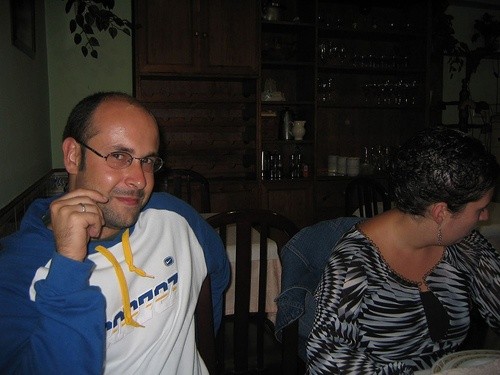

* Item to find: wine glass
[359,79,422,106]
[318,39,347,65]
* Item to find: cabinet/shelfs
[132,0,500,246]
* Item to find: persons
[303,128,500,375]
[0,92,232,375]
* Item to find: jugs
[288,120,307,140]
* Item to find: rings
[80,203,86,213]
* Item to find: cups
[328,143,394,176]
[352,51,410,69]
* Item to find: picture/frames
[9,0,37,58]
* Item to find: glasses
[76,140,162,173]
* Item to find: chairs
[153,168,392,375]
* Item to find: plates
[431,350,500,373]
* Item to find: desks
[199,211,282,327]
[354,199,500,256]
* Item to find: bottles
[279,107,294,140]
[261,151,302,181]
